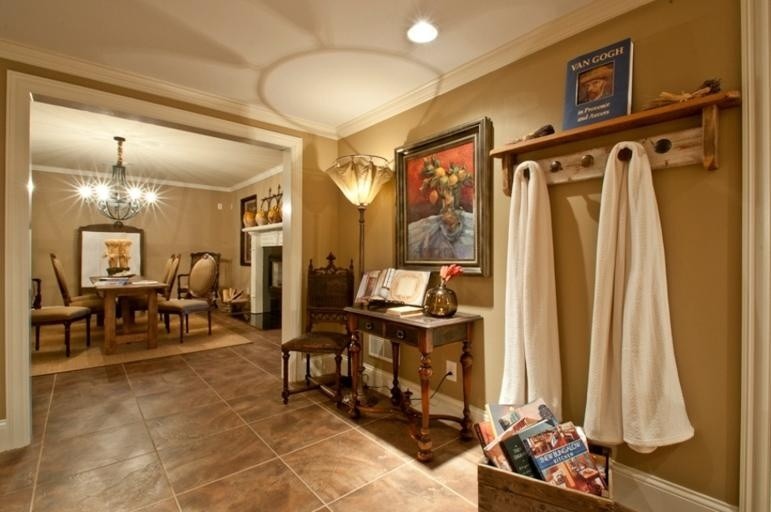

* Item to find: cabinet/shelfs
[344,305,482,462]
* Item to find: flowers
[438,265,464,286]
[418,155,475,207]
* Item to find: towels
[500,160,565,422]
[582,141,693,457]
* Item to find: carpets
[25,303,254,377]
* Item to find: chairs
[125,255,184,334]
[49,252,115,341]
[131,253,176,297]
[29,278,92,358]
[280,251,354,412]
[155,253,219,345]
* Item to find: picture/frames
[393,117,492,277]
[78,225,144,288]
[240,195,256,266]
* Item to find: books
[474,397,609,504]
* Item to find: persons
[579,66,613,105]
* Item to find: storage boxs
[478,439,617,512]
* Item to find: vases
[423,284,458,318]
[439,197,463,241]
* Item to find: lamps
[80,136,155,226]
[323,154,393,301]
